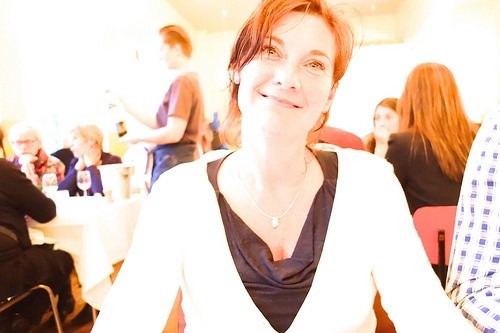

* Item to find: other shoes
[58,295,75,314]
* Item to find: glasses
[11,139,37,145]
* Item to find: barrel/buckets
[98,164,134,202]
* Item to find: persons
[0,124,100,333]
[8,123,65,190]
[384,63,481,291]
[108,24,206,194]
[57,123,124,197]
[445,107,500,333]
[360,98,400,158]
[90,0,479,333]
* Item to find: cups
[42,173,59,197]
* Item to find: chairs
[0,223,65,333]
[414,206,465,286]
[176,125,363,333]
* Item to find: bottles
[106,88,128,138]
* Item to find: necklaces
[237,149,308,229]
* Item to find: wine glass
[77,170,92,204]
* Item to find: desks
[28,196,147,323]
